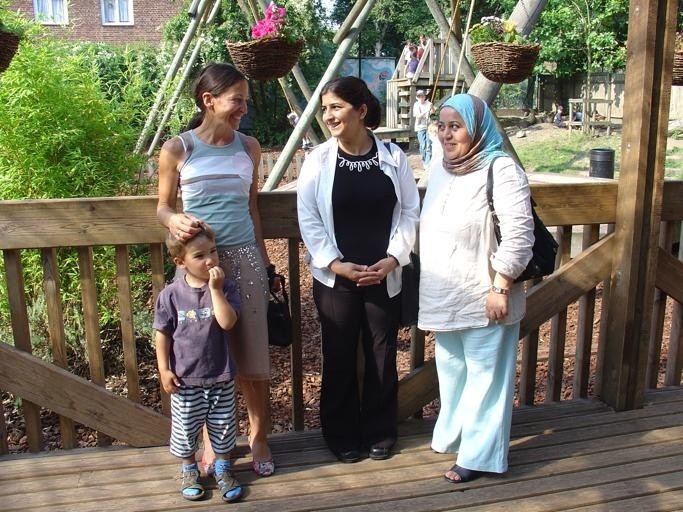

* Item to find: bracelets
[492,285,510,295]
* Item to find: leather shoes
[368,441,392,461]
[337,447,361,464]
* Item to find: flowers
[467,16,536,45]
[249,0,299,46]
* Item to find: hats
[416,90,426,96]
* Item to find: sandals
[213,469,245,503]
[181,468,206,500]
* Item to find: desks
[568,98,613,136]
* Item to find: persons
[404,33,429,76]
[416,93,535,482]
[151,223,244,501]
[553,106,566,128]
[157,63,281,480]
[412,89,435,170]
[296,75,421,463]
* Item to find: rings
[177,230,182,237]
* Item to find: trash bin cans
[589,149,614,179]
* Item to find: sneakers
[247,435,275,478]
[201,457,216,476]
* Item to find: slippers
[444,464,483,484]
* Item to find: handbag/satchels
[267,300,293,349]
[400,251,419,328]
[492,204,559,284]
